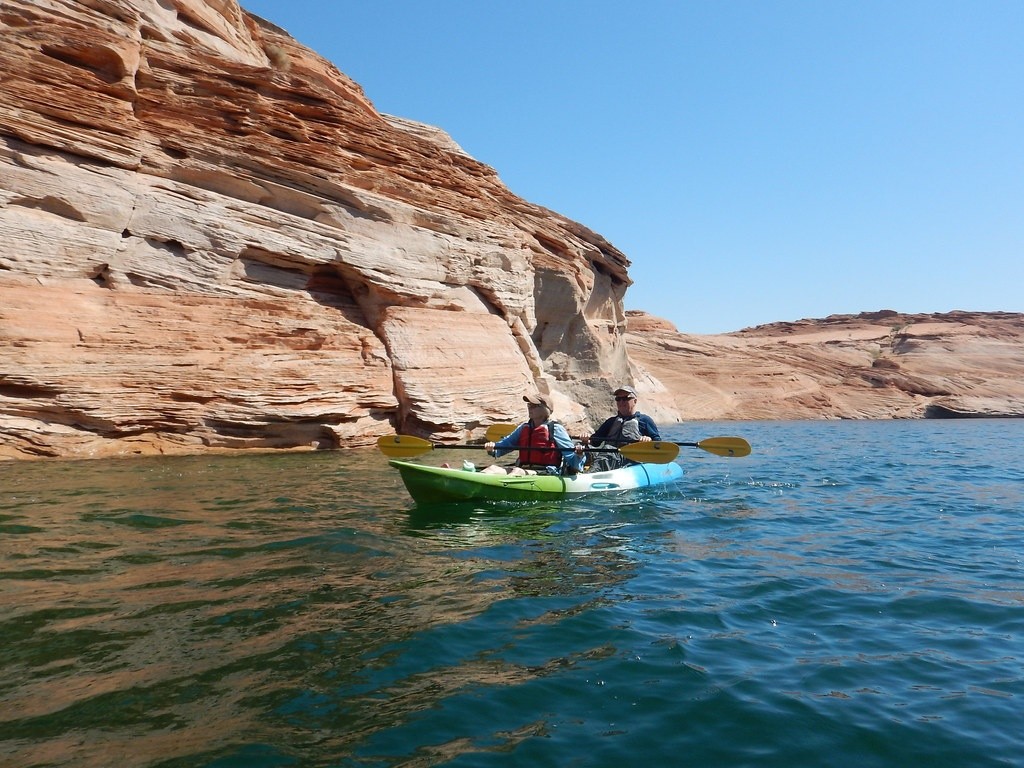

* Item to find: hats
[614,385,637,396]
[523,393,553,411]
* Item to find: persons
[578,386,662,474]
[480,393,586,474]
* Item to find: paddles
[485,423,751,458]
[376,435,681,466]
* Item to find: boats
[388,459,684,504]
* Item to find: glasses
[615,395,631,401]
[527,403,538,409]
